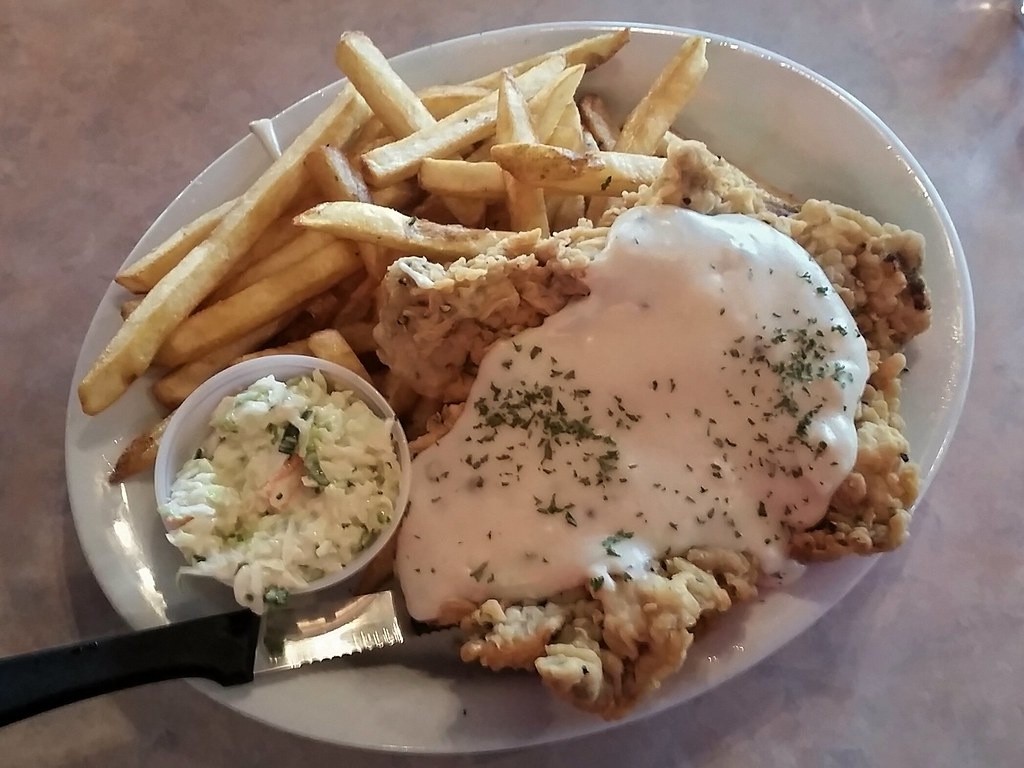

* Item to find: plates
[63,20,974,755]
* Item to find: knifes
[0,589,464,728]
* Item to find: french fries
[78,29,710,483]
